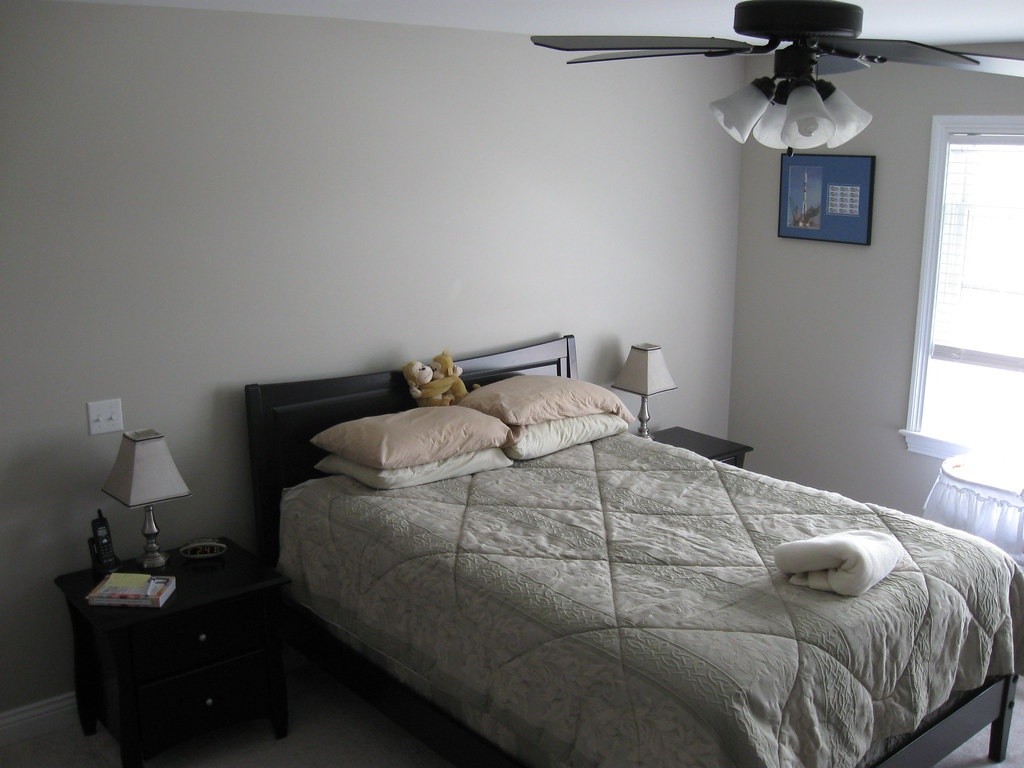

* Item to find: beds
[242,335,1024,768]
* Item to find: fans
[529,0,1024,77]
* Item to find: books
[88,572,175,608]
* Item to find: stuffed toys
[403,352,481,406]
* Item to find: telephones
[89,508,119,570]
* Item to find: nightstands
[651,426,754,469]
[50,539,299,768]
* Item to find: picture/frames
[779,153,875,246]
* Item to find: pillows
[314,447,513,489]
[310,405,517,474]
[457,375,635,427]
[504,412,628,460]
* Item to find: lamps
[708,46,872,150]
[611,342,678,440]
[101,428,193,568]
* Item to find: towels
[774,530,904,597]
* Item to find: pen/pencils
[146,577,155,596]
